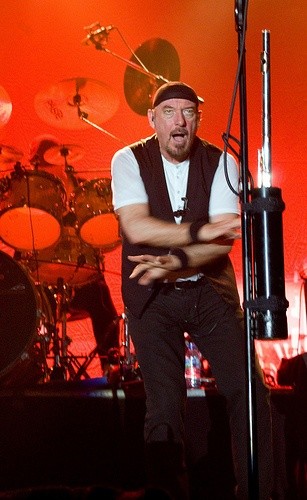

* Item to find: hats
[153,81,200,108]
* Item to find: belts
[157,275,209,291]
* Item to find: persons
[12,137,120,373]
[107,78,282,500]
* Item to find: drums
[0,169,66,251]
[68,176,121,250]
[24,216,104,290]
[0,248,46,384]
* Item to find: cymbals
[44,144,85,167]
[123,36,181,116]
[0,85,13,127]
[32,77,120,131]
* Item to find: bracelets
[169,246,189,273]
[188,220,208,244]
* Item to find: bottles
[184,342,201,393]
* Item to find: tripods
[40,286,92,379]
